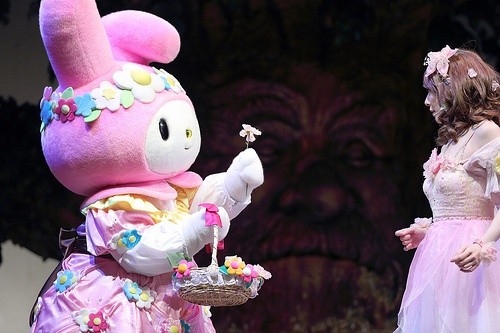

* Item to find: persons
[391,46,500,333]
[186,3,423,333]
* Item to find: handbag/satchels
[172,208,263,305]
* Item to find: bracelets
[472,240,498,266]
[410,214,434,231]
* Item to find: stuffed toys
[30,0,264,333]
